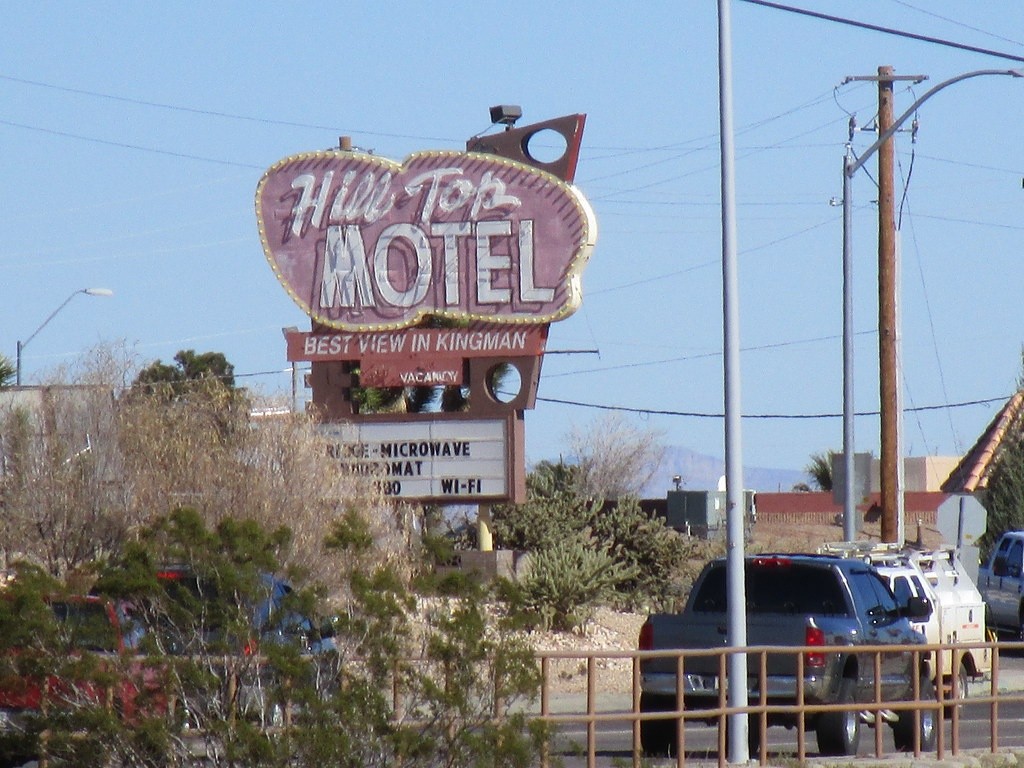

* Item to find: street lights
[17,288,114,385]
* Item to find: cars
[0,588,185,737]
[87,559,344,728]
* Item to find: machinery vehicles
[817,541,998,719]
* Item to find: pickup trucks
[637,552,940,758]
[977,532,1024,640]
